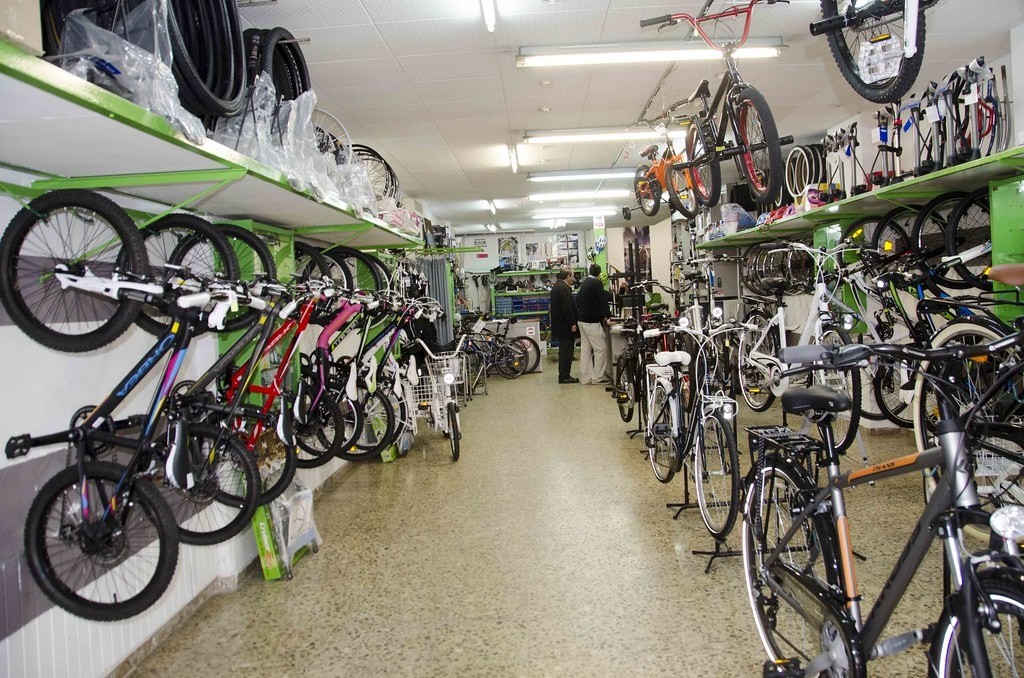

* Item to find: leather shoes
[559,376,578,383]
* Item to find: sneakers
[597,380,612,384]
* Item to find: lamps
[516,36,790,68]
[487,199,497,215]
[527,189,631,201]
[527,166,636,182]
[522,126,687,144]
[487,223,498,234]
[508,144,519,176]
[481,0,498,33]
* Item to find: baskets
[412,352,467,405]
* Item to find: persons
[603,281,630,303]
[550,267,580,384]
[575,263,613,385]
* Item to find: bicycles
[3,190,265,622]
[115,0,1024,678]
